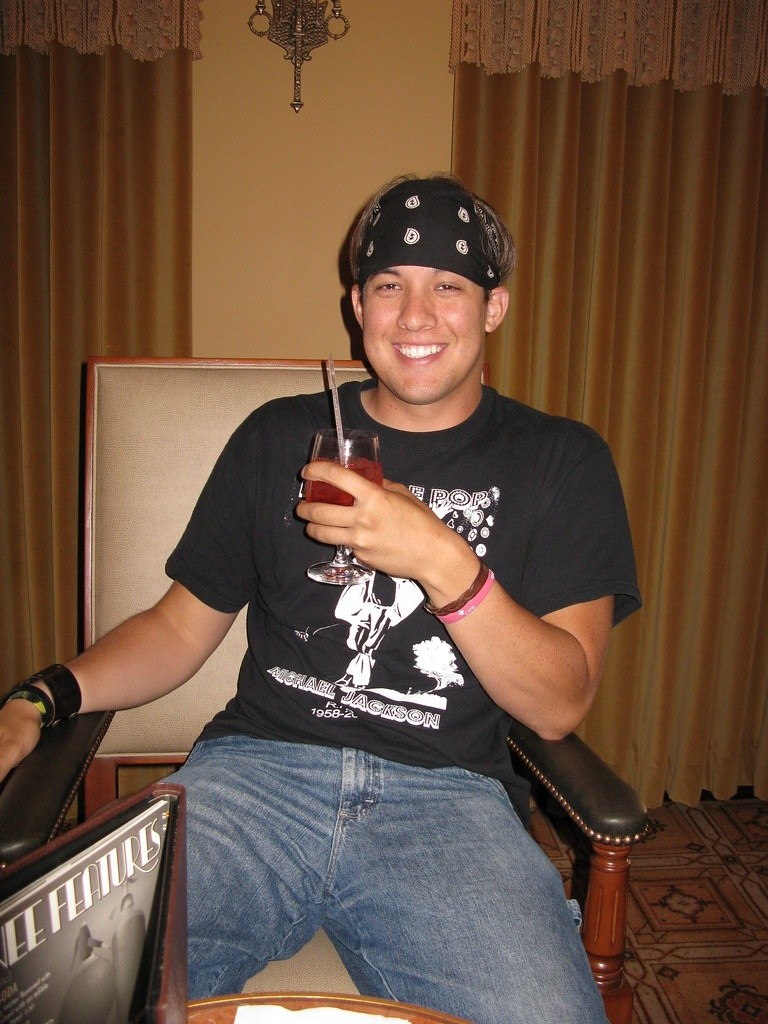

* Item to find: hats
[349,180,501,290]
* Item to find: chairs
[0,358,654,1024]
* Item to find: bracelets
[436,569,495,624]
[3,663,82,729]
[424,561,489,616]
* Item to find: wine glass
[305,429,383,585]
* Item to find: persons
[0,174,645,1023]
